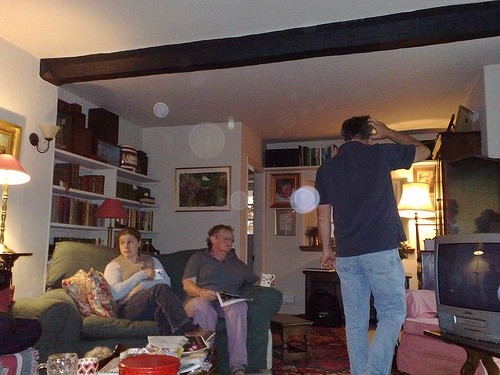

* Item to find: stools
[271,315,314,365]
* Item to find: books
[47,163,155,260]
[297,144,337,167]
[213,289,255,308]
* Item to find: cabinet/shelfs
[44,148,160,293]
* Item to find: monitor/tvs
[434,232,500,343]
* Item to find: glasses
[219,233,234,242]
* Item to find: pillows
[61,267,118,317]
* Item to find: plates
[145,339,213,373]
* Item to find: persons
[276,180,294,201]
[183,225,275,375]
[312,115,431,375]
[103,227,215,347]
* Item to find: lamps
[94,199,129,248]
[29,124,61,153]
[0,153,30,253]
[397,182,435,290]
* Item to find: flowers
[398,241,416,261]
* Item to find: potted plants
[304,226,319,247]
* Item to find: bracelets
[130,291,135,296]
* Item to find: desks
[301,267,412,328]
[423,329,500,375]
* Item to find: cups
[260,273,275,288]
[77,358,99,375]
[34,352,78,375]
[118,354,181,375]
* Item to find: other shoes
[231,365,246,375]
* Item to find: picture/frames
[269,172,300,208]
[174,166,231,212]
[274,208,297,236]
[408,160,441,209]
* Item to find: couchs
[397,290,500,375]
[11,241,283,375]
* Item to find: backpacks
[307,289,338,327]
